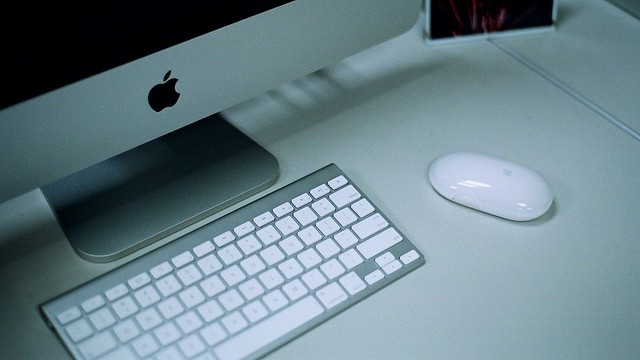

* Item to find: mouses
[428,151,554,222]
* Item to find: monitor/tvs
[1,0,425,263]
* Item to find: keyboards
[37,162,426,359]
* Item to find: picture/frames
[422,0,559,45]
